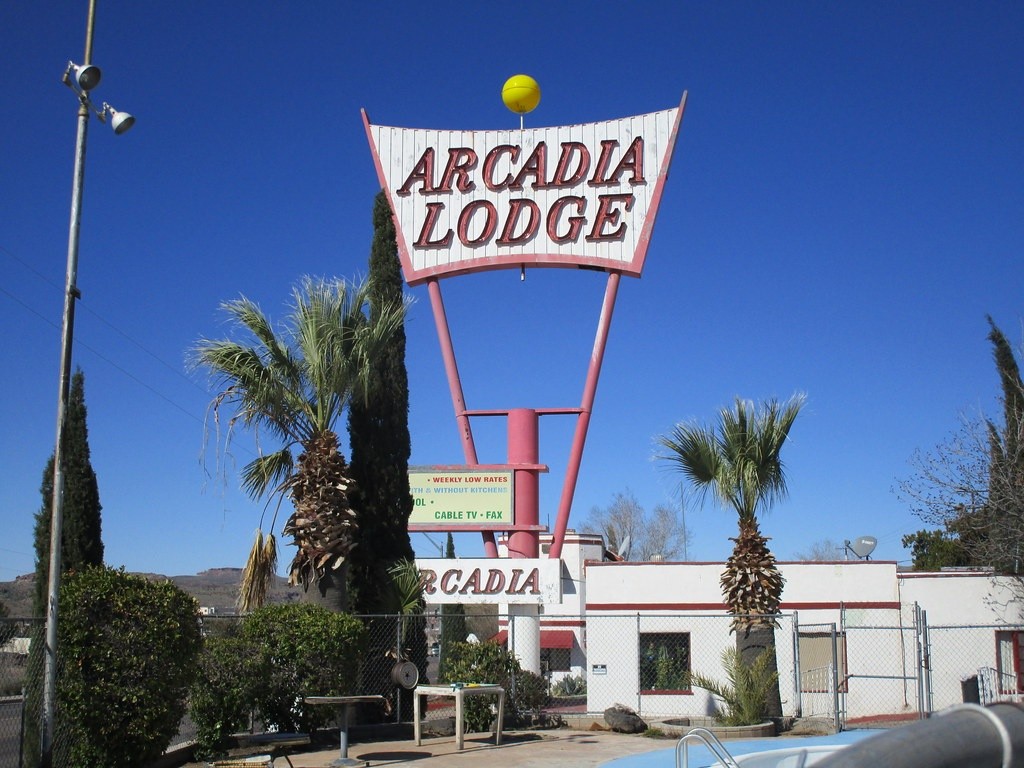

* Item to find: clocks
[391,662,420,689]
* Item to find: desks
[306,694,386,767]
[413,684,505,750]
[247,733,310,768]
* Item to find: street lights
[37,1,137,768]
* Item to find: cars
[429,642,440,657]
